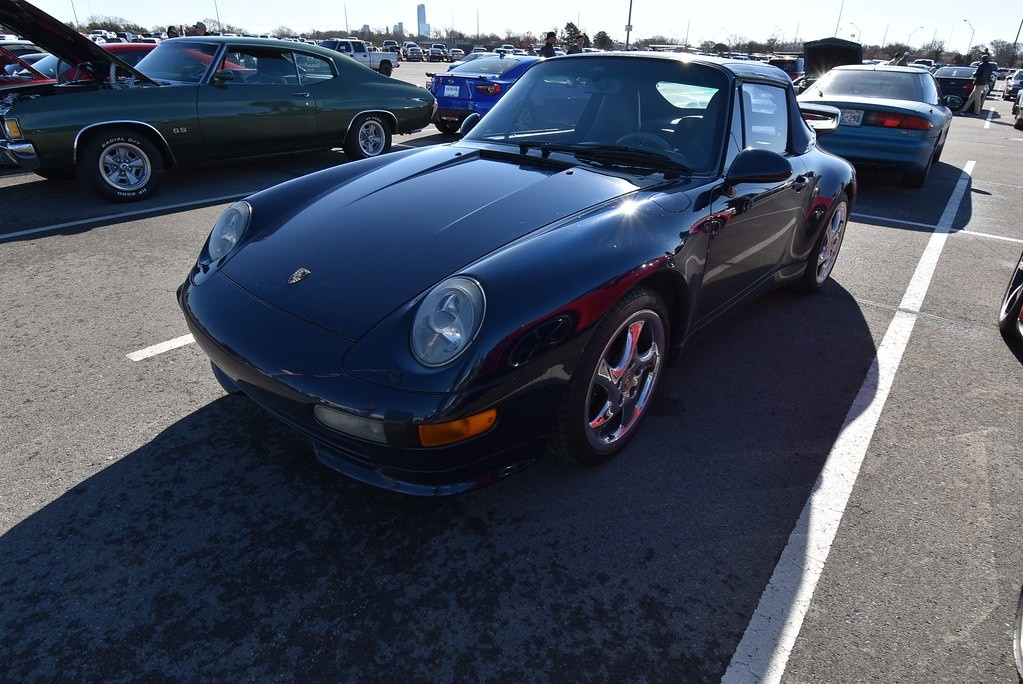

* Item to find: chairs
[583,89,641,143]
[687,89,752,167]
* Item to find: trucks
[803,37,862,89]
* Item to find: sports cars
[176,51,856,500]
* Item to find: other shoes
[971,112,979,117]
[954,111,964,115]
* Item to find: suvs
[501,44,515,52]
[970,62,998,91]
[473,47,487,53]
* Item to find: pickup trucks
[306,40,400,78]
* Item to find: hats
[544,32,556,40]
[193,22,206,30]
[904,52,909,56]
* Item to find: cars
[0,43,246,93]
[81,30,169,44]
[998,67,1023,129]
[493,48,507,55]
[349,37,402,61]
[0,35,51,75]
[932,66,989,111]
[553,47,566,56]
[0,0,438,207]
[402,41,465,62]
[583,48,601,52]
[513,49,527,56]
[216,32,321,69]
[795,62,963,192]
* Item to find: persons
[897,52,910,66]
[528,44,537,56]
[539,32,557,58]
[566,35,586,54]
[167,26,179,38]
[956,55,992,117]
[888,52,900,65]
[193,21,214,56]
